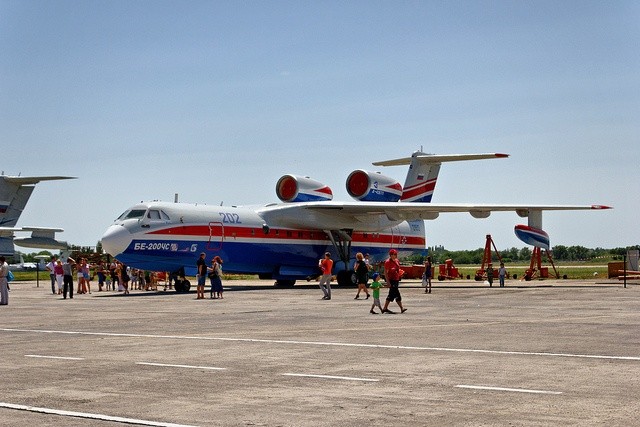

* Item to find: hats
[373,273,380,281]
[389,249,399,254]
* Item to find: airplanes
[100,146,614,293]
[0,171,79,256]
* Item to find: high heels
[366,294,370,299]
[354,294,359,299]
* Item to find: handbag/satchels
[208,271,218,278]
[7,271,14,281]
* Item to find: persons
[45,255,57,294]
[53,258,65,295]
[362,253,373,288]
[196,253,208,299]
[75,255,178,295]
[482,263,494,287]
[383,249,407,314]
[354,252,370,300]
[497,263,508,286]
[0,256,9,305]
[423,256,432,293]
[369,273,385,314]
[62,256,75,298]
[319,252,333,300]
[210,259,216,299]
[207,256,224,299]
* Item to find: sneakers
[326,297,331,300]
[381,309,386,314]
[370,310,375,314]
[401,309,407,313]
[322,296,327,299]
[384,308,391,312]
